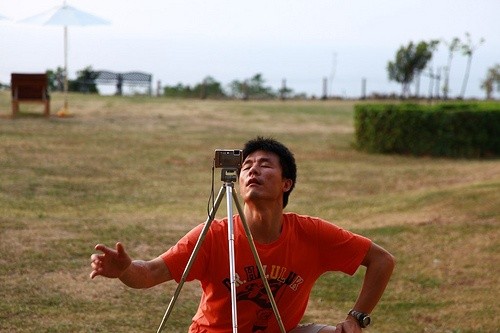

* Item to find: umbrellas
[14,0,114,118]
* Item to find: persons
[88,133,398,333]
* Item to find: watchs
[346,308,371,328]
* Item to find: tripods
[156,182,287,333]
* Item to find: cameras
[214,150,243,169]
